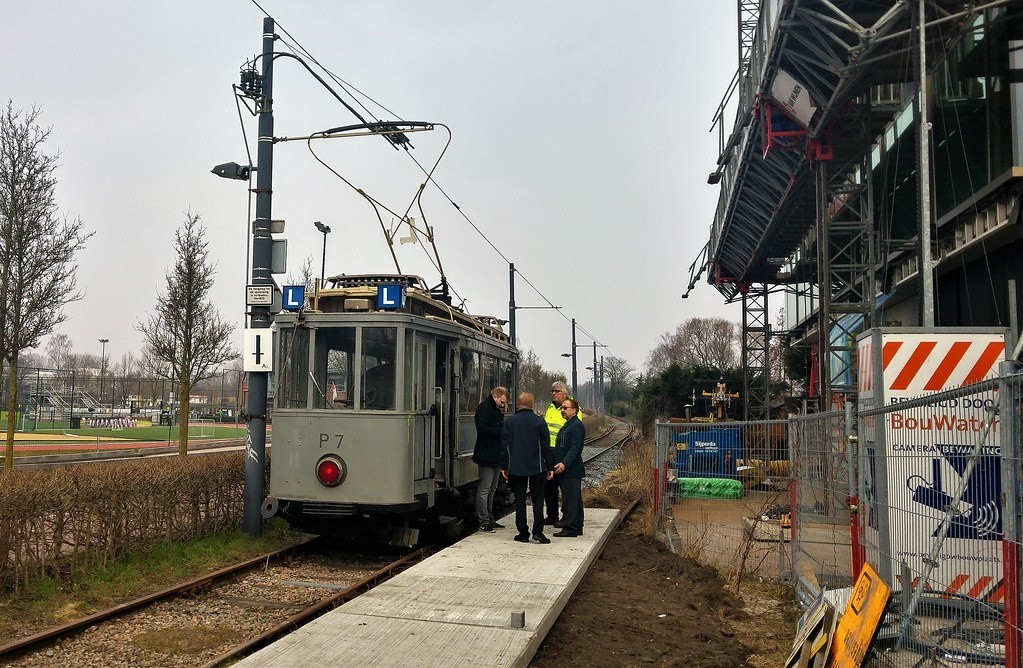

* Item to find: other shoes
[514,534,529,542]
[554,520,564,528]
[563,527,584,535]
[552,531,577,537]
[543,516,560,525]
[533,533,550,543]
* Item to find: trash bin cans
[71,417,81,429]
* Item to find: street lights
[491,262,517,348]
[586,341,599,410]
[99,338,109,408]
[213,17,278,536]
[312,220,332,289]
[559,318,579,401]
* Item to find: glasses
[560,406,576,410]
[550,389,564,394]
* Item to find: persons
[472,387,509,533]
[542,381,583,527]
[553,399,586,538]
[499,392,555,544]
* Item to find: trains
[270,119,521,548]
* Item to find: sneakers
[489,522,506,530]
[479,525,495,533]
